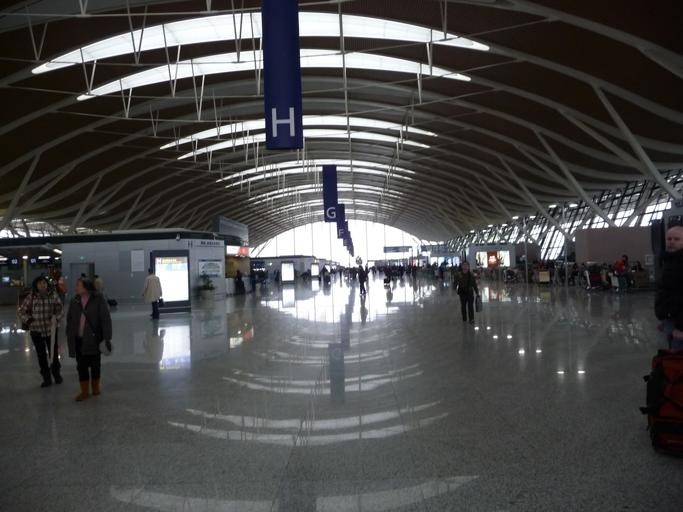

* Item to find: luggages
[105,294,117,307]
[638,334,682,460]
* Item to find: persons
[234,270,243,295]
[16,276,66,388]
[355,265,367,295]
[359,295,368,325]
[64,276,112,403]
[140,267,164,319]
[40,271,105,308]
[249,270,256,291]
[452,260,480,325]
[386,288,393,303]
[501,250,645,295]
[141,320,165,376]
[301,259,463,286]
[652,224,683,351]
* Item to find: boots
[51,362,63,385]
[92,378,100,395]
[75,379,89,401]
[40,367,52,388]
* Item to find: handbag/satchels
[100,340,111,355]
[22,310,31,331]
[159,299,164,307]
[475,295,482,313]
